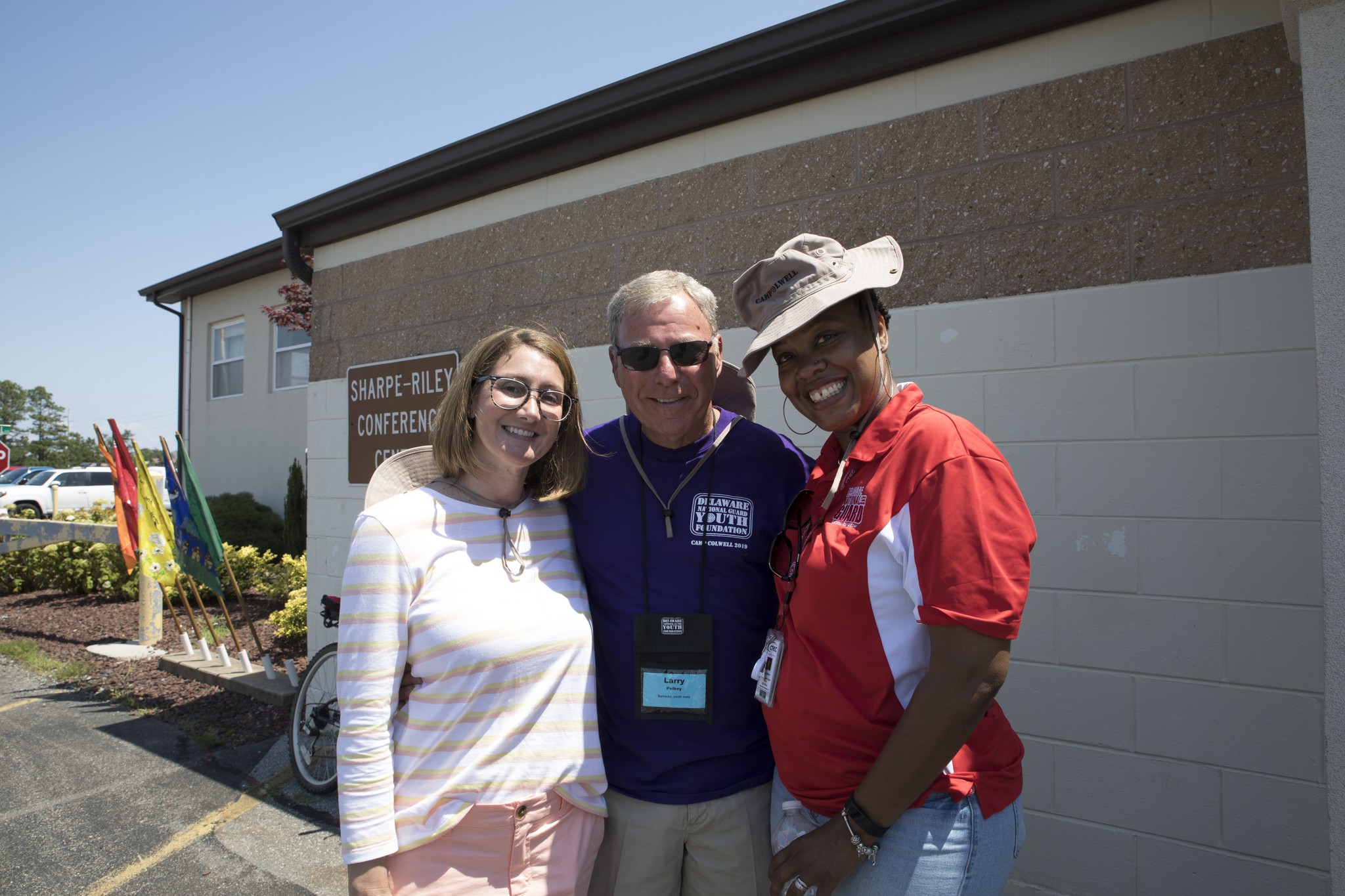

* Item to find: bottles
[771,801,817,896]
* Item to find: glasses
[612,333,715,371]
[768,489,814,582]
[471,374,580,423]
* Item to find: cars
[0,466,56,487]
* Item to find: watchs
[843,790,890,839]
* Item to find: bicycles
[286,599,342,798]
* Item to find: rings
[794,876,810,892]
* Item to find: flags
[90,420,233,599]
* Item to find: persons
[733,233,1038,896]
[558,272,820,896]
[335,325,609,896]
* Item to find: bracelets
[840,809,880,866]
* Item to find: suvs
[147,466,176,510]
[0,464,121,520]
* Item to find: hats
[712,359,757,422]
[732,233,904,376]
